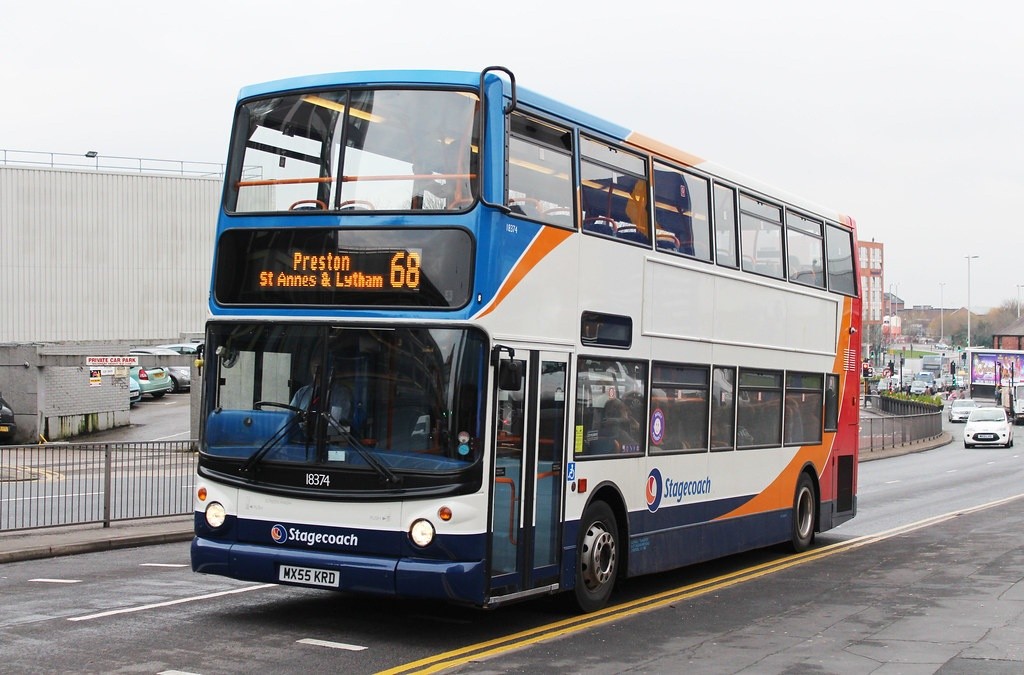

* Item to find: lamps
[86,151,97,157]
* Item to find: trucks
[1002,382,1024,425]
[874,355,965,397]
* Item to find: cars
[963,407,1014,449]
[130,348,190,394]
[128,375,142,404]
[948,399,982,423]
[934,343,953,351]
[130,366,172,398]
[156,343,203,359]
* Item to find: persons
[287,354,351,436]
[996,386,1002,405]
[865,375,966,408]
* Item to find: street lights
[888,279,901,343]
[967,254,979,350]
[938,282,948,341]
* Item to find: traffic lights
[951,362,955,374]
[952,377,956,386]
[862,363,873,378]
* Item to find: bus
[194,66,865,614]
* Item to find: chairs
[589,433,622,453]
[290,199,375,211]
[448,198,817,286]
